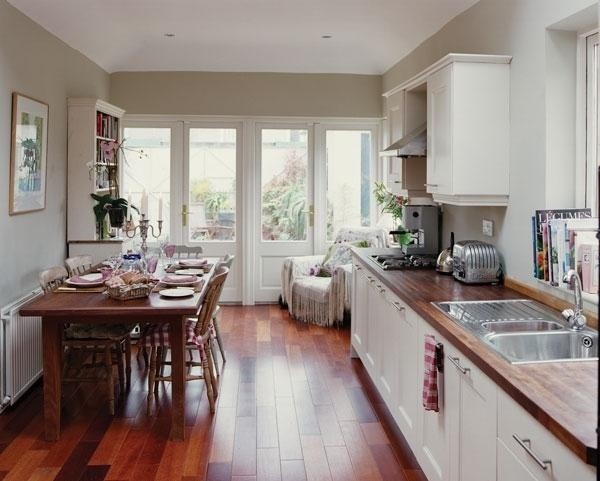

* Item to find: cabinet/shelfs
[418,315,497,481]
[66,95,126,240]
[376,277,418,463]
[350,254,376,388]
[496,386,597,481]
[384,89,444,206]
[425,62,509,207]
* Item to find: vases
[109,208,124,227]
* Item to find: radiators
[0,285,44,407]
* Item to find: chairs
[136,244,235,416]
[279,225,391,328]
[39,252,137,416]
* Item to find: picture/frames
[9,91,50,215]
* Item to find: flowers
[85,137,150,221]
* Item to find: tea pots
[435,231,454,273]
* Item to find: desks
[67,231,135,277]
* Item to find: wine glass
[102,239,176,273]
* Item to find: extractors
[379,118,426,159]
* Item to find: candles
[126,187,163,222]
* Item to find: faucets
[562,268,583,312]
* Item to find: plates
[156,286,197,299]
[63,273,105,288]
[160,259,208,286]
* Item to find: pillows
[316,230,371,276]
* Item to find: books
[532,207,598,294]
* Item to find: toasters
[451,241,501,284]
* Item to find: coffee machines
[401,197,439,256]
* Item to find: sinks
[485,331,599,365]
[481,320,564,333]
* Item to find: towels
[421,334,443,412]
